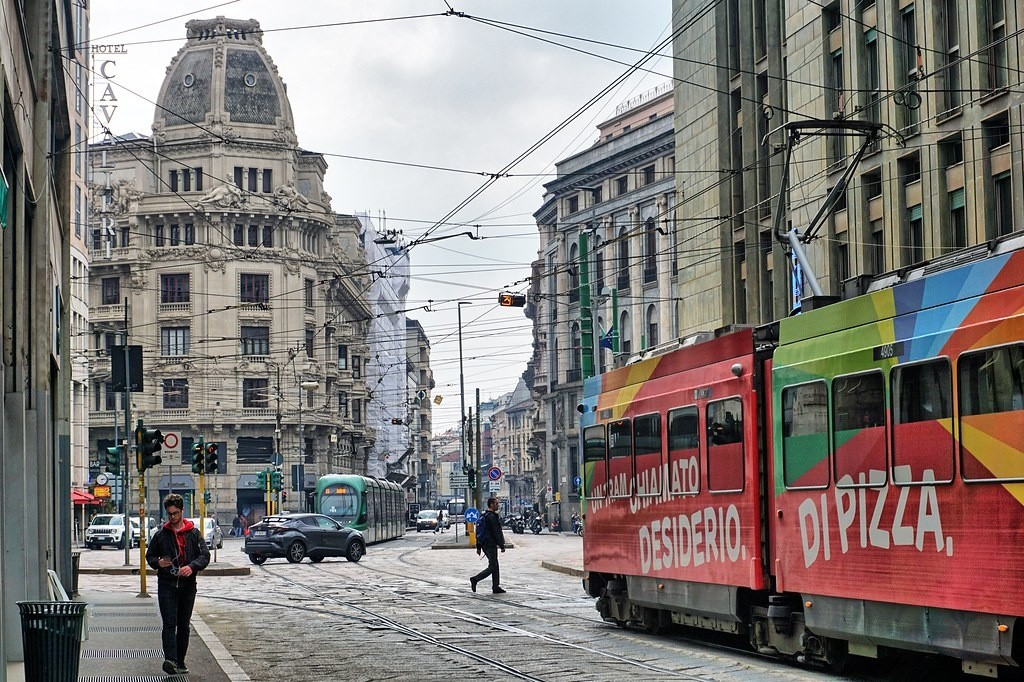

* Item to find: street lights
[457,301,473,536]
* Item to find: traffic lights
[205,442,218,472]
[278,473,285,492]
[467,468,475,487]
[255,471,265,490]
[191,442,204,474]
[282,491,287,503]
[141,428,163,468]
[500,295,513,306]
[105,447,120,476]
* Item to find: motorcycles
[509,513,541,535]
[576,521,584,536]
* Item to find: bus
[576,230,1023,682]
[309,474,405,544]
[447,499,466,525]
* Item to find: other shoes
[177,664,189,673]
[493,587,506,594]
[162,660,178,675]
[470,576,477,592]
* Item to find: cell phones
[162,556,171,561]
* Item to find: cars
[85,514,134,550]
[130,517,158,548]
[186,518,224,550]
[416,510,451,532]
[245,514,366,565]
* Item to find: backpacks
[475,511,493,545]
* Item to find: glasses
[166,509,182,518]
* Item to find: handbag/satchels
[244,528,249,535]
[228,528,235,535]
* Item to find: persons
[433,510,444,534]
[233,514,247,536]
[146,494,210,674]
[88,179,95,218]
[160,518,165,525]
[470,498,506,593]
[523,510,536,523]
[195,171,240,206]
[406,508,415,528]
[572,511,579,533]
[90,509,97,522]
[275,180,309,208]
[118,179,132,214]
